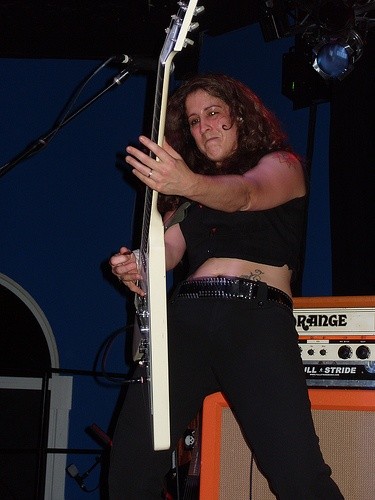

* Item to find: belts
[168,276,294,311]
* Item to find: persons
[109,74,344,500]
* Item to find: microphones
[119,54,175,76]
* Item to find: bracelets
[132,249,140,269]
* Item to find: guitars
[132,0,205,451]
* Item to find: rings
[148,168,153,179]
